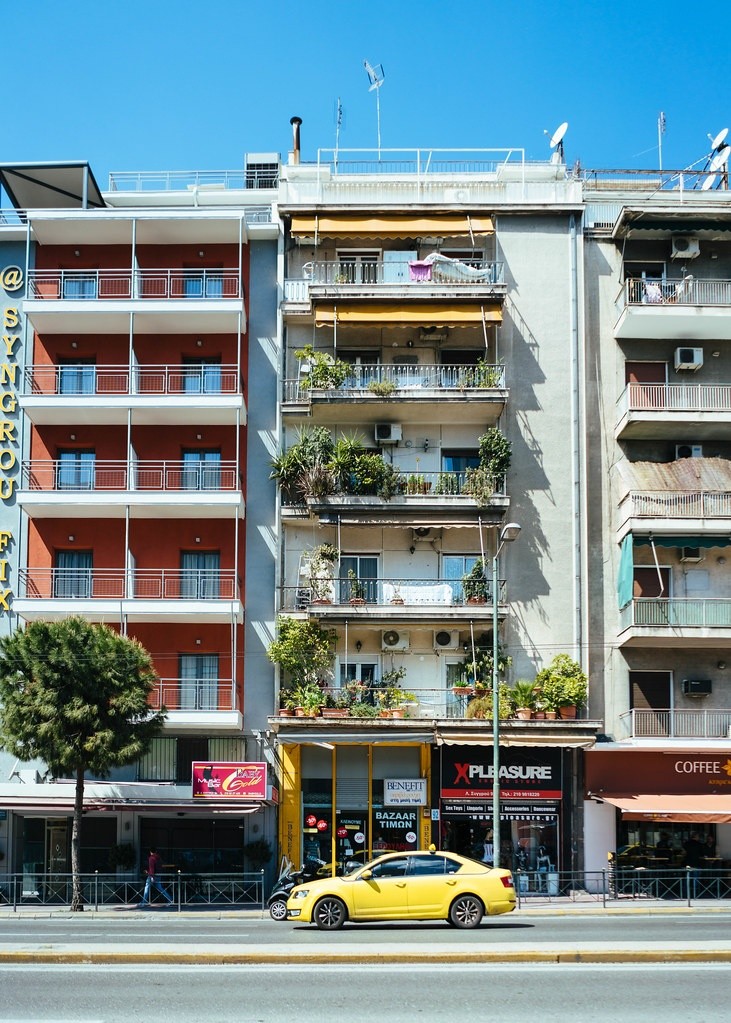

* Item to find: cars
[317,850,397,875]
[617,844,656,856]
[668,831,706,846]
[287,851,517,931]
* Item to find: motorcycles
[268,861,312,920]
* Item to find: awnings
[276,725,436,745]
[315,303,504,328]
[611,205,731,243]
[316,512,503,529]
[437,727,597,751]
[320,619,499,631]
[616,529,731,614]
[587,791,731,824]
[290,216,496,238]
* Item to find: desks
[617,844,724,894]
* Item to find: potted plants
[266,613,587,719]
[294,345,505,390]
[269,421,514,511]
[247,836,273,872]
[302,543,492,606]
[336,272,348,284]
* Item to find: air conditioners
[674,347,704,370]
[417,237,445,245]
[682,678,712,698]
[670,236,701,259]
[413,528,441,542]
[381,630,410,651]
[419,326,448,341]
[295,237,323,245]
[676,444,703,460]
[375,423,403,442]
[433,629,460,650]
[680,547,706,562]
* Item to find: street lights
[491,523,522,868]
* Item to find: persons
[682,832,721,898]
[139,847,174,905]
[534,846,551,892]
[514,838,533,892]
[655,833,671,898]
[479,827,493,867]
[303,837,321,859]
